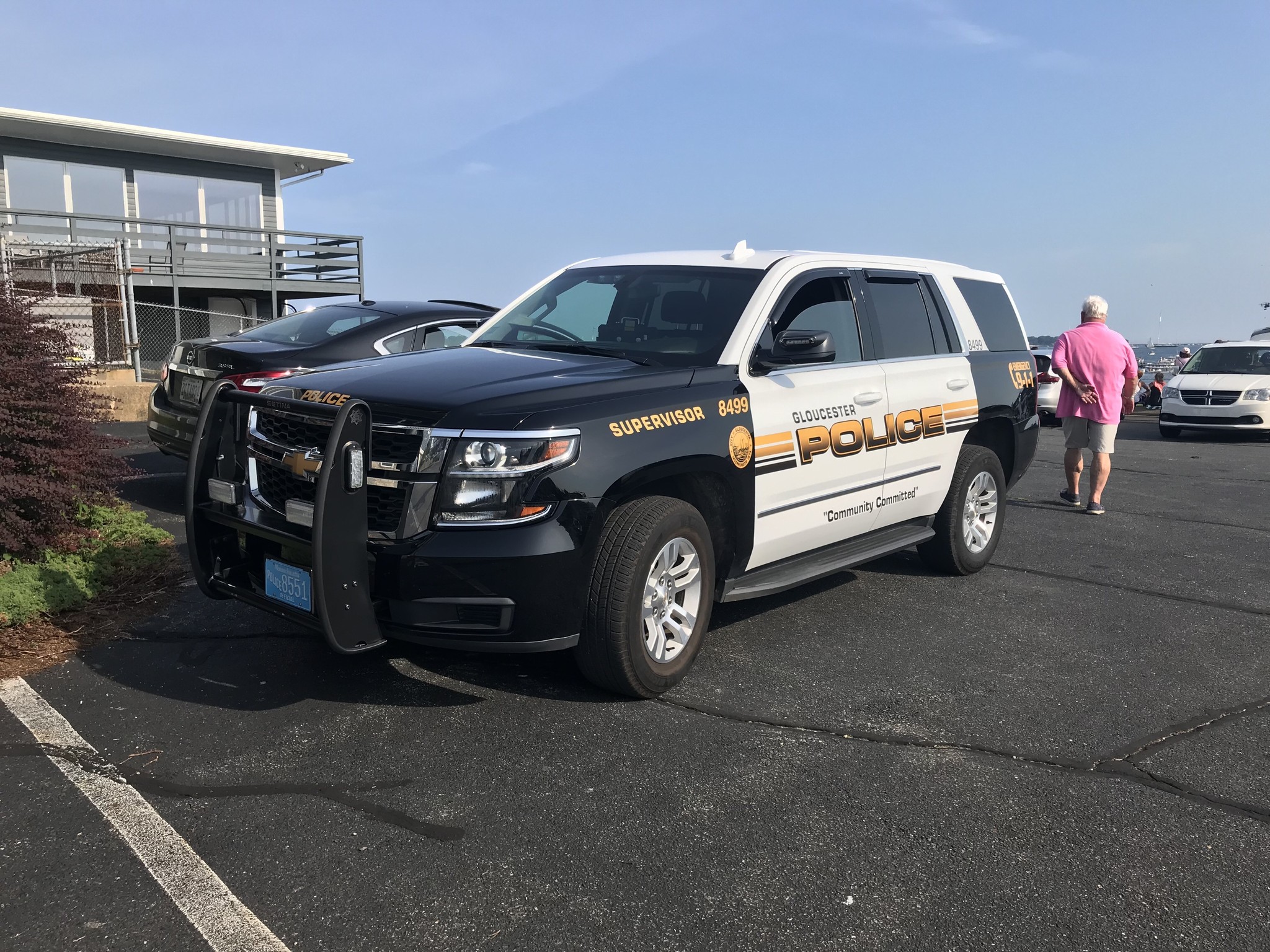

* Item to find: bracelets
[1122,394,1134,400]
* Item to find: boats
[1148,351,1155,355]
[1146,354,1192,374]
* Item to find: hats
[1180,347,1191,354]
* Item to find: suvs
[185,241,1042,699]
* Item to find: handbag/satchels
[1172,365,1180,376]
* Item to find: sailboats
[1146,338,1155,349]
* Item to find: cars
[1159,327,1270,442]
[1030,349,1125,425]
[146,298,586,463]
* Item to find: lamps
[293,161,304,173]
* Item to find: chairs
[149,242,188,273]
[652,290,709,340]
[1204,352,1270,374]
[424,328,445,350]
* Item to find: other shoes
[1150,406,1162,409]
[1145,404,1153,409]
[1059,488,1082,505]
[1086,501,1106,515]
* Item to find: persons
[1174,347,1192,371]
[1134,370,1151,404]
[1145,373,1166,409]
[1051,295,1138,514]
